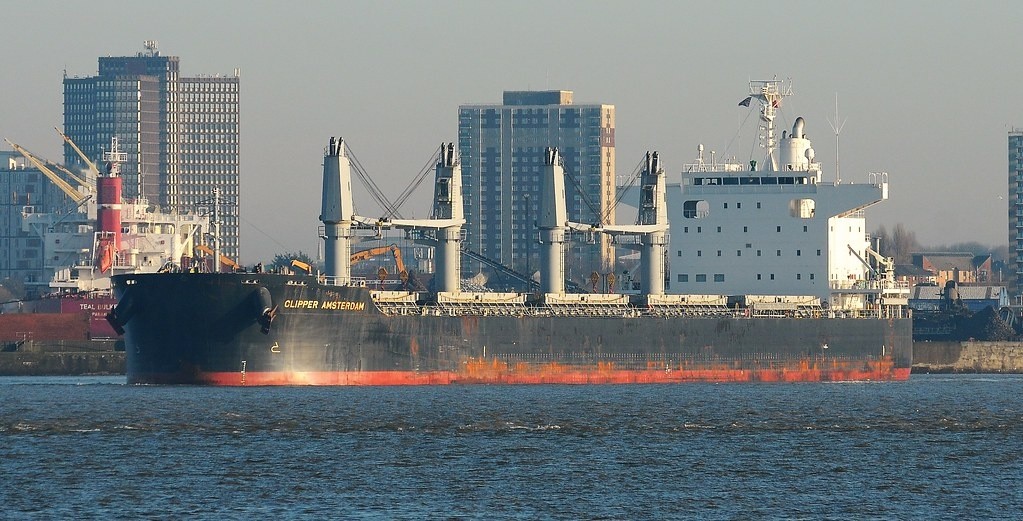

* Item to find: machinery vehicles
[193,245,239,273]
[316,245,409,292]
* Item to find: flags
[772,99,778,108]
[95,237,116,274]
[738,97,754,107]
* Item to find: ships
[105,269,916,389]
